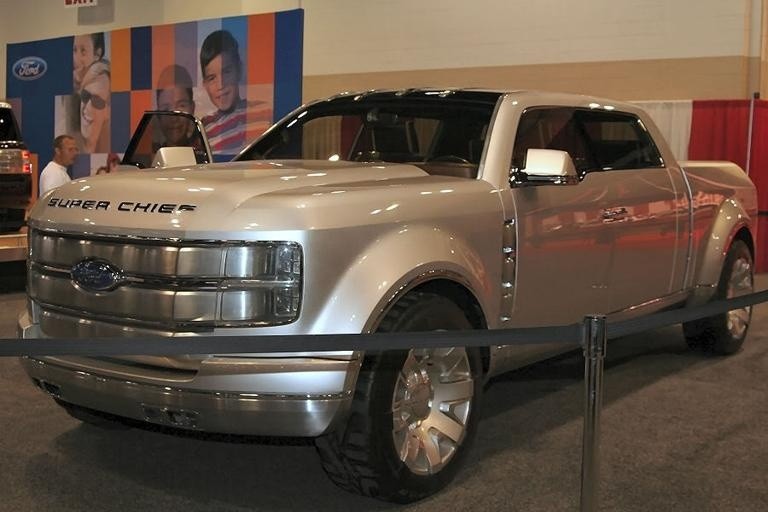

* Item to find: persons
[50,33,110,152]
[156,64,209,163]
[39,135,78,198]
[97,152,120,175]
[198,30,274,156]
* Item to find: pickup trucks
[17,86,757,504]
[0,101,38,234]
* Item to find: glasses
[78,88,108,110]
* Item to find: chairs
[435,116,491,163]
[353,116,427,161]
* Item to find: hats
[106,153,120,162]
